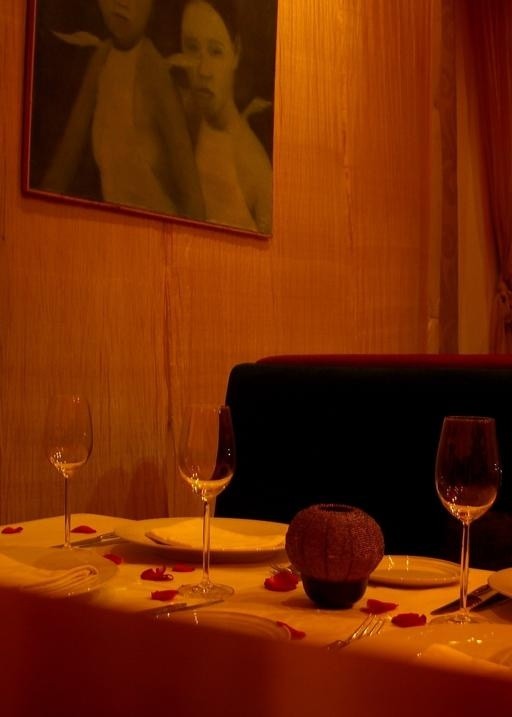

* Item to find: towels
[146,519,284,549]
[0,553,97,596]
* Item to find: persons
[38,0,206,222]
[177,0,271,232]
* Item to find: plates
[368,555,461,591]
[1,541,117,603]
[487,567,512,599]
[109,508,294,567]
[151,604,293,655]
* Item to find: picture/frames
[22,0,279,240]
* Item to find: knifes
[430,584,499,617]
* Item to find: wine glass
[173,403,236,601]
[428,417,499,624]
[42,391,94,557]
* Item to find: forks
[316,611,385,659]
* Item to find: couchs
[211,355,512,572]
